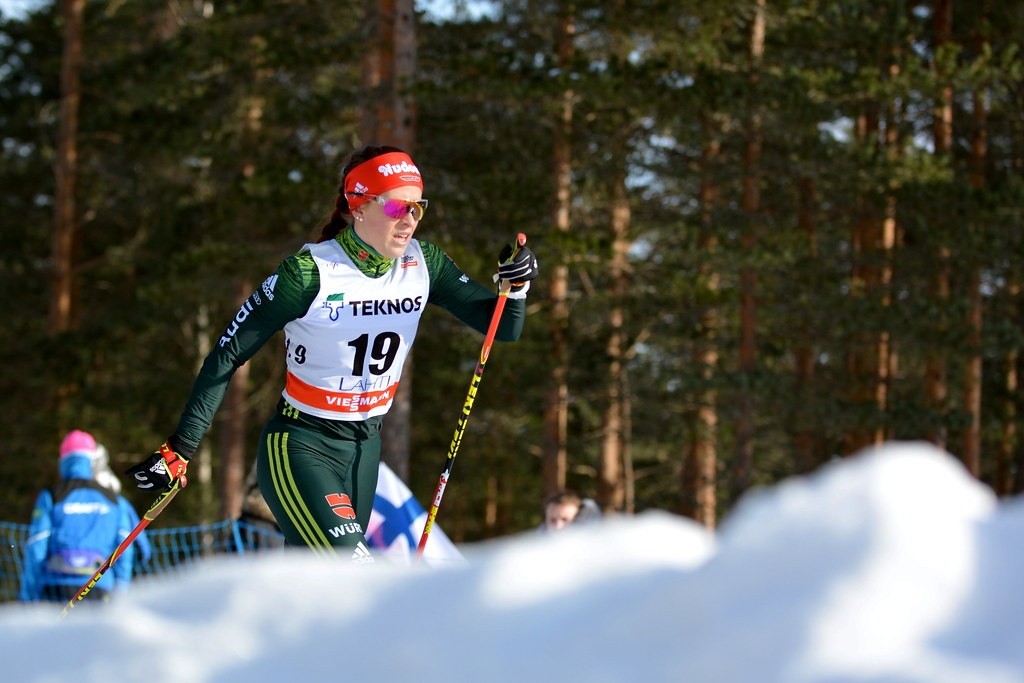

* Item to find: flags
[363,459,463,560]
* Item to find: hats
[60,430,97,457]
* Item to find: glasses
[348,192,428,221]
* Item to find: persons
[137,146,539,570]
[543,492,581,530]
[230,457,285,554]
[20,429,150,607]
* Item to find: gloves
[124,439,189,491]
[492,244,538,300]
